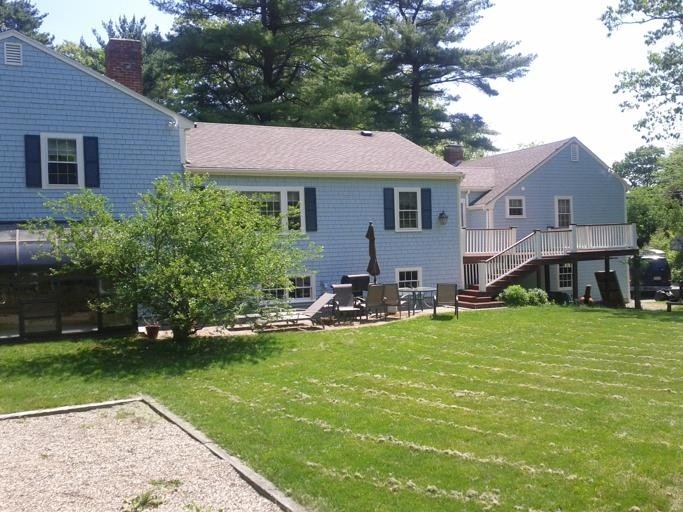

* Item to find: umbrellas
[365,221,380,318]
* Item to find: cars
[630,255,672,300]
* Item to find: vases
[145,325,159,338]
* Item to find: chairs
[226,282,459,335]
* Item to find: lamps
[438,210,447,225]
